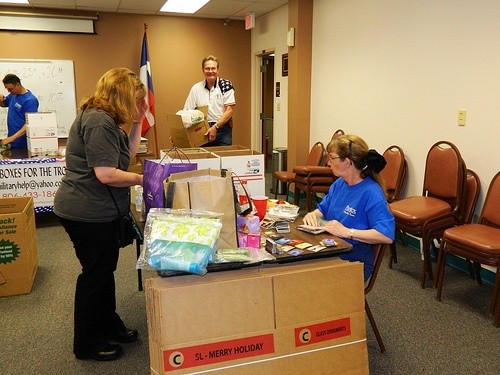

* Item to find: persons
[302,135,395,284]
[53,67,145,359]
[183,55,236,148]
[0,74,39,148]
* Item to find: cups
[251,196,268,222]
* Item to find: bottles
[238,180,250,205]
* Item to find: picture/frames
[282,53,288,76]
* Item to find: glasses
[326,155,342,162]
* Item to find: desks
[0,158,67,213]
[129,204,355,291]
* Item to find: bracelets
[214,124,220,131]
[351,228,354,239]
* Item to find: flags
[139,33,155,138]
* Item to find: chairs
[275,130,500,352]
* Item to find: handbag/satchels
[116,209,144,248]
[143,146,198,216]
[163,168,239,249]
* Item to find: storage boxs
[25,112,58,158]
[0,197,39,297]
[160,144,266,200]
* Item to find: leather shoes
[74,343,122,360]
[110,327,139,343]
